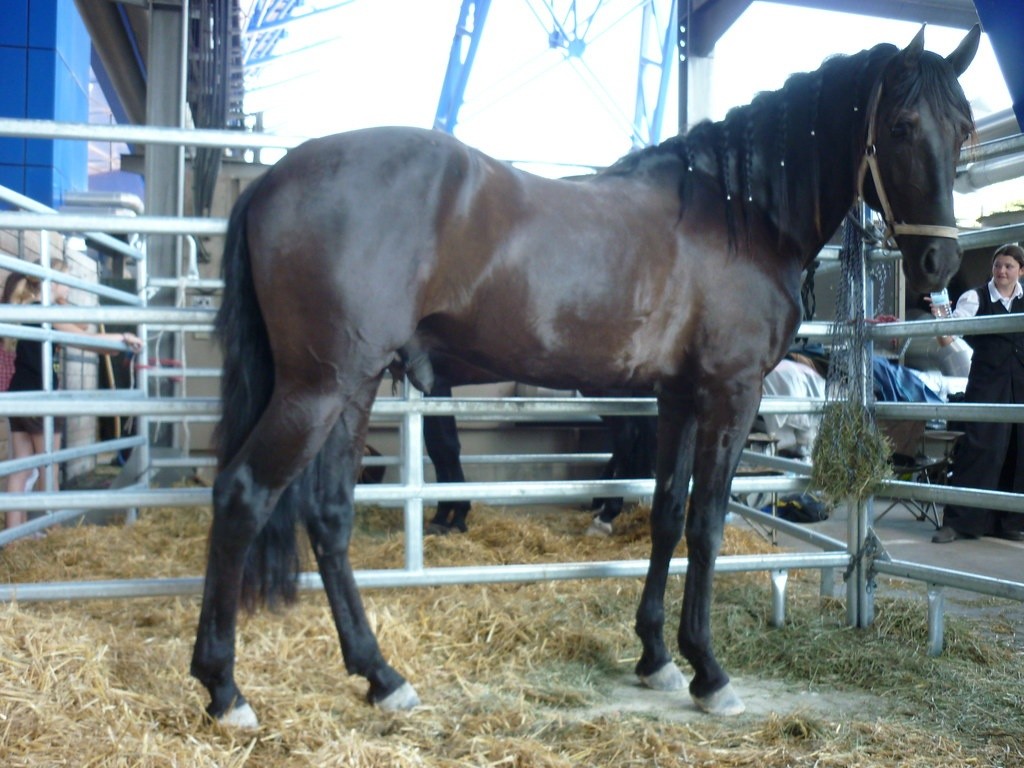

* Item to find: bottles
[930,287,955,339]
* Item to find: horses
[187,22,981,733]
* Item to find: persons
[923,245,1024,542]
[0,256,143,531]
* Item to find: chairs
[872,419,964,531]
[724,432,780,548]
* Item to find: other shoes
[985,530,1024,541]
[932,525,964,543]
